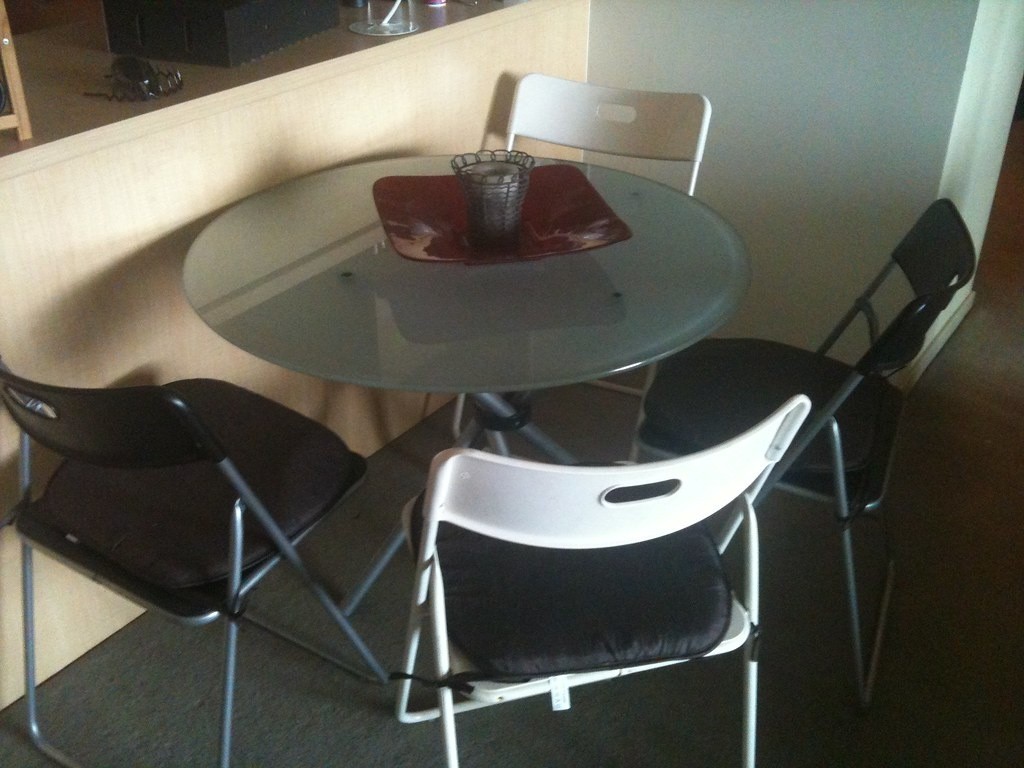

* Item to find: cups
[367,0,413,31]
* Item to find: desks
[180,151,751,618]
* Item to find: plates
[373,162,632,264]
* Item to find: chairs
[0,356,387,768]
[454,71,712,466]
[393,393,813,768]
[631,198,976,718]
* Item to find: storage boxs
[100,0,341,70]
